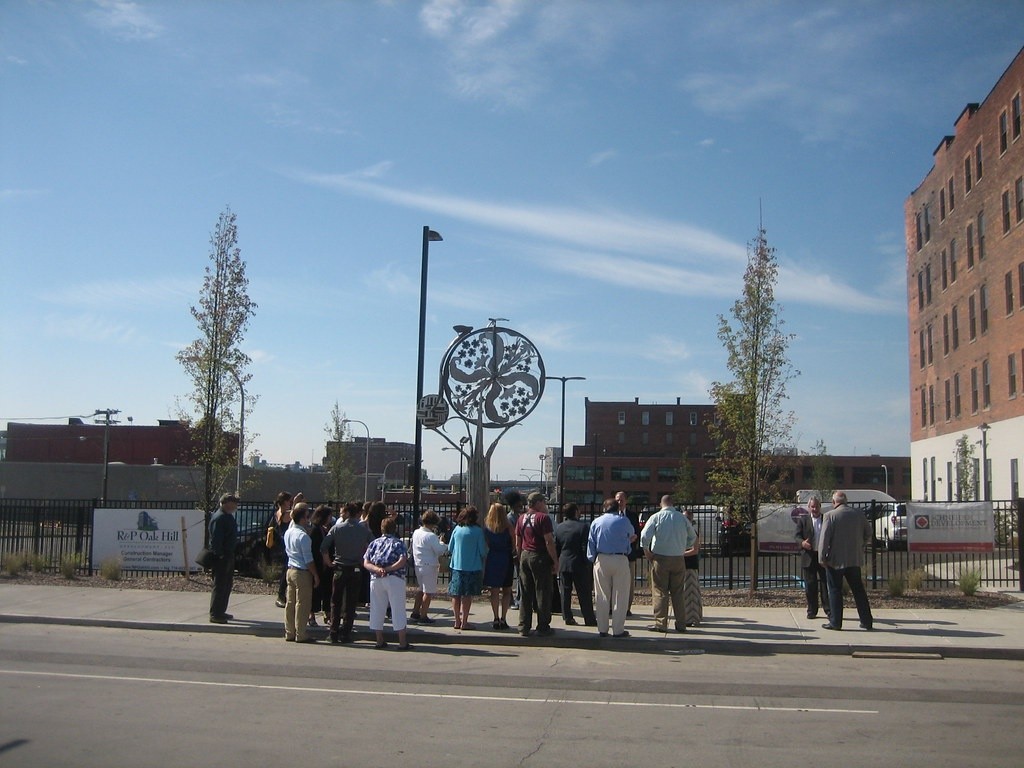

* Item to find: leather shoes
[860,624,871,629]
[822,624,840,631]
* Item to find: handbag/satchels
[266,526,276,548]
[195,549,214,569]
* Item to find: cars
[233,489,908,576]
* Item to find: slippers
[399,643,413,650]
[376,644,386,648]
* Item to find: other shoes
[223,613,233,619]
[275,612,359,643]
[410,613,419,621]
[452,604,597,636]
[419,617,435,625]
[626,611,631,615]
[613,631,629,637]
[807,613,814,618]
[648,624,663,632]
[676,628,685,632]
[599,633,607,636]
[275,599,285,608]
[210,617,227,624]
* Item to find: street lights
[186,356,244,498]
[977,422,991,501]
[342,420,369,503]
[521,468,547,496]
[441,436,469,503]
[545,377,585,522]
[881,465,888,494]
[382,459,423,504]
[414,225,444,527]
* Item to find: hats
[220,494,240,504]
[528,492,549,504]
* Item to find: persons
[409,509,448,625]
[818,490,873,629]
[446,505,488,629]
[640,495,699,632]
[682,510,703,627]
[389,509,456,545]
[209,492,241,624]
[504,491,563,637]
[483,503,516,629]
[553,502,598,627]
[265,491,403,644]
[609,492,639,615]
[793,494,831,620]
[363,517,415,650]
[587,498,635,637]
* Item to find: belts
[611,553,626,555]
